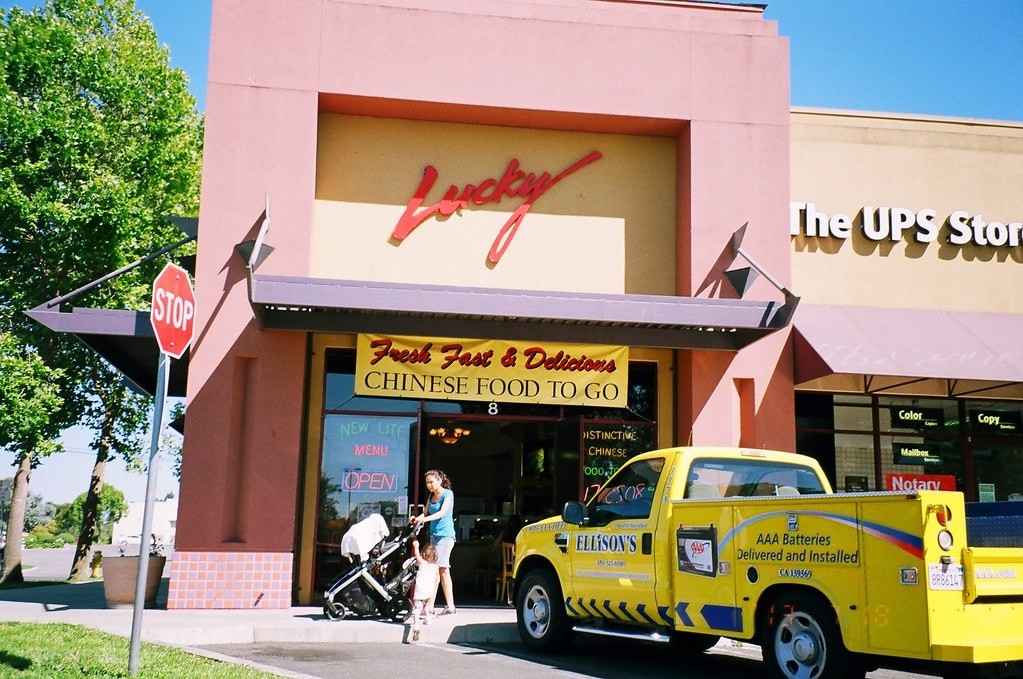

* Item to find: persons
[413,539,439,640]
[408,470,456,617]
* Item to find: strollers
[320,512,425,623]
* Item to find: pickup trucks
[508,445,1023,679]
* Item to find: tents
[250,269,801,331]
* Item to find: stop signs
[152,262,196,357]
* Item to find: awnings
[792,304,1022,385]
[21,307,189,398]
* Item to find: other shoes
[412,626,421,641]
[423,619,430,625]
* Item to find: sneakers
[419,609,437,619]
[437,605,457,618]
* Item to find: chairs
[495,542,516,604]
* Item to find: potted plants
[102,533,166,609]
